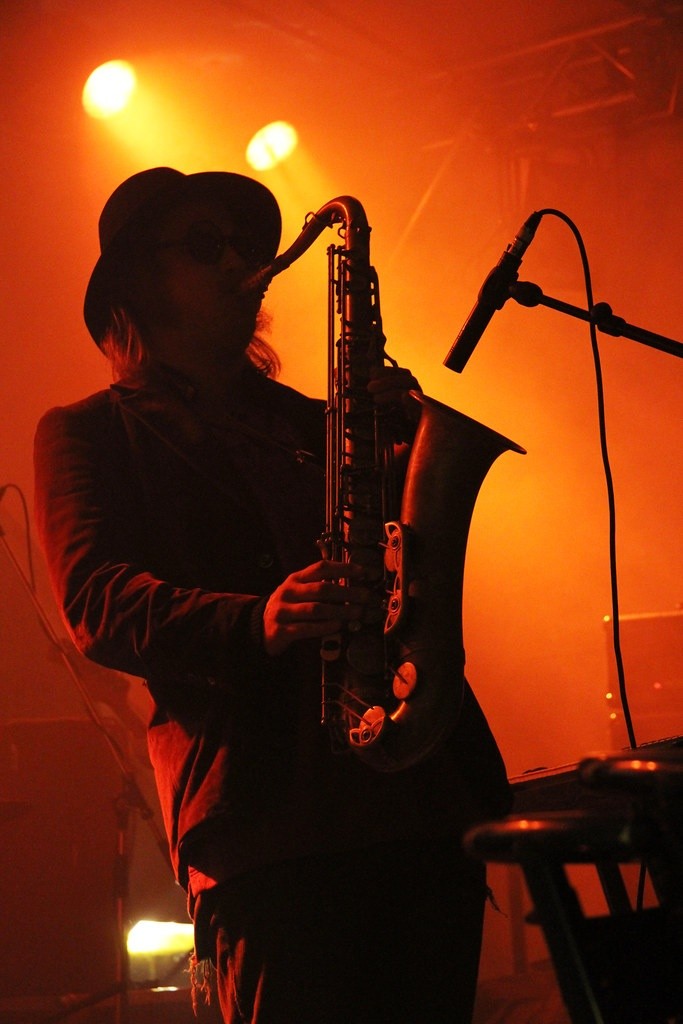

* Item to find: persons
[31,164,530,1024]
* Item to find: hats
[83,167,282,357]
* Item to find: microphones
[443,210,541,374]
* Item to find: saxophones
[247,194,528,777]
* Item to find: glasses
[134,219,271,269]
